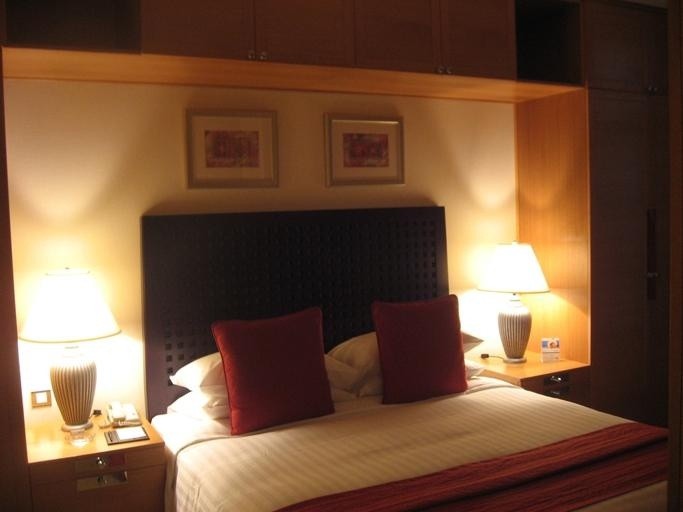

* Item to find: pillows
[370,296,469,405]
[167,348,363,396]
[327,332,480,368]
[354,365,484,398]
[165,389,360,422]
[208,306,335,434]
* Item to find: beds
[140,206,683,512]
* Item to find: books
[103,425,150,447]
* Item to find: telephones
[105,402,140,427]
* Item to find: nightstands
[24,406,165,512]
[492,347,591,407]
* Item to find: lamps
[474,241,552,361]
[17,269,122,431]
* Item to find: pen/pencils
[108,431,113,441]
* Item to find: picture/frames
[184,106,278,189]
[323,111,404,186]
